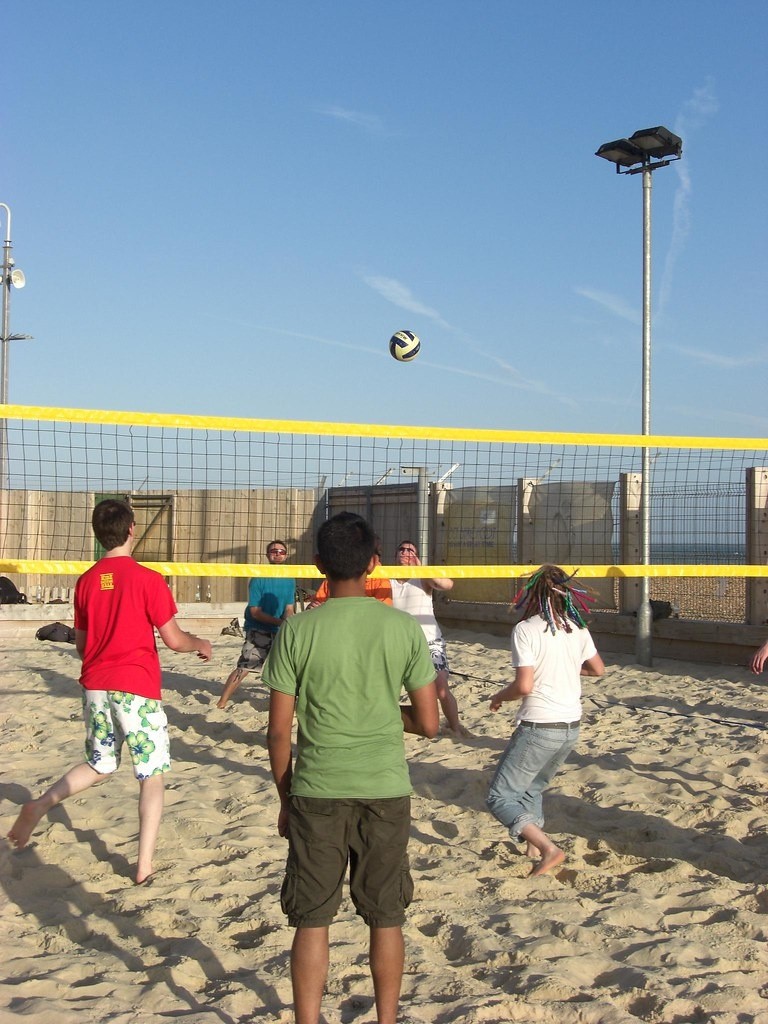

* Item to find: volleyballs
[388,329,421,363]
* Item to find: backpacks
[0,575,32,605]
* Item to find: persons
[7,499,211,885]
[749,639,768,676]
[387,541,473,739]
[485,564,605,879]
[306,535,394,610]
[216,539,297,710]
[260,510,440,1024]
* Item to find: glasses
[397,546,415,553]
[269,548,286,555]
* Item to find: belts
[521,721,581,729]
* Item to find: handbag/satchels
[35,621,70,641]
